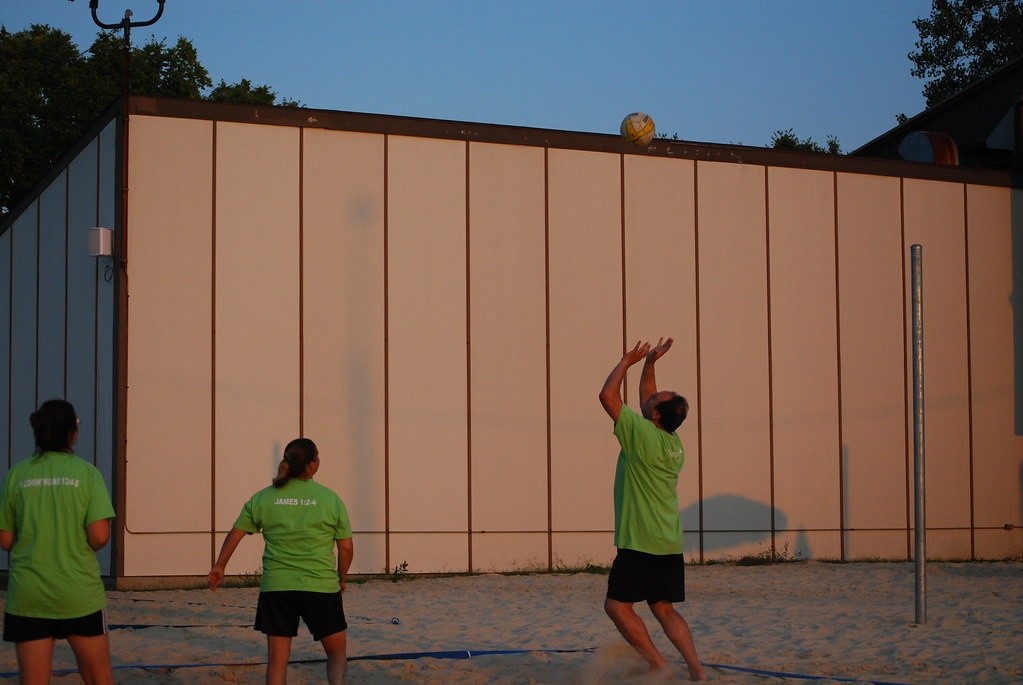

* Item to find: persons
[599,336,708,682]
[208,438,354,685]
[0,399,116,685]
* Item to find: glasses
[74,418,80,427]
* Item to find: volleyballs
[619,112,656,148]
